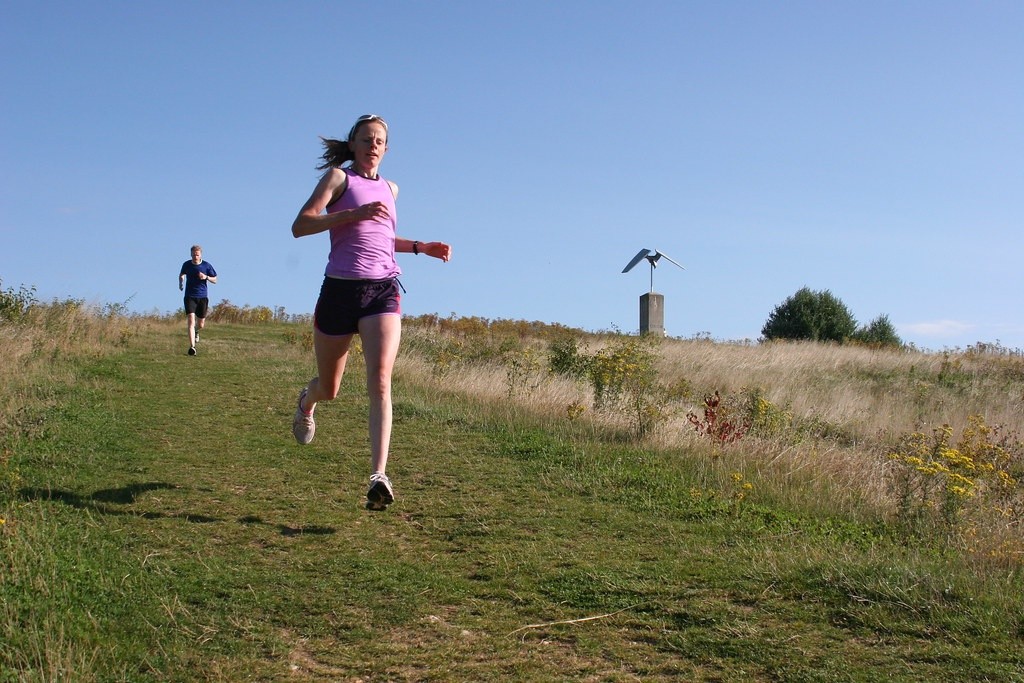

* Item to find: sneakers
[365,472,395,512]
[292,387,317,445]
[188,346,197,356]
[195,330,200,342]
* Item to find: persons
[179,245,217,356]
[290,116,451,511]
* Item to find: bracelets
[413,241,419,255]
[206,276,209,279]
